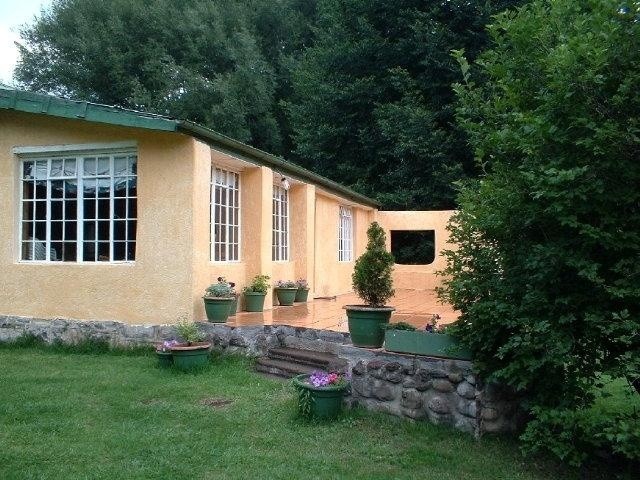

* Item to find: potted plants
[344,221,397,350]
[169,311,212,371]
[202,272,272,323]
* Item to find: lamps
[272,165,290,191]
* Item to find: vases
[384,328,475,360]
[156,350,172,369]
[295,374,349,419]
[276,286,309,306]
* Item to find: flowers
[423,313,445,335]
[274,277,308,288]
[156,341,178,352]
[307,372,338,388]
